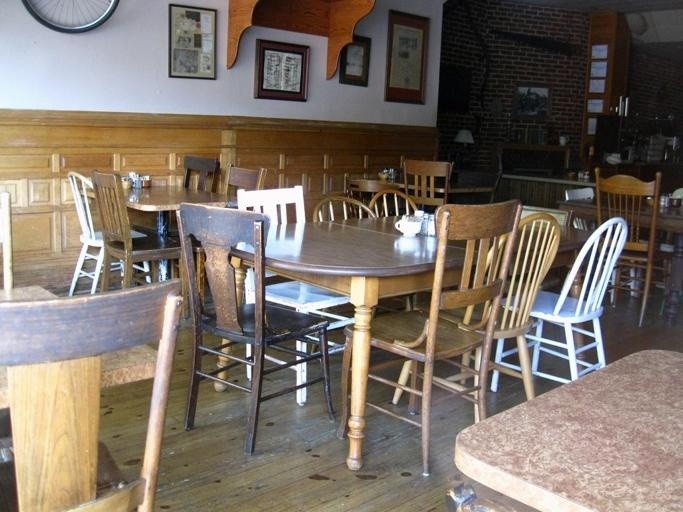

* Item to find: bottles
[419,236,439,259]
[664,147,669,161]
[414,209,443,237]
[644,192,681,209]
[119,171,151,190]
[567,169,592,181]
[376,166,401,179]
[588,146,595,156]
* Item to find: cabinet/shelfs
[581,10,630,145]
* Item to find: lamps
[454,130,474,150]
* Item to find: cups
[615,106,619,112]
[395,214,422,237]
[392,235,420,256]
[559,136,569,146]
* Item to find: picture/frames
[339,34,371,87]
[511,80,552,124]
[384,9,430,105]
[168,6,217,79]
[254,38,310,102]
